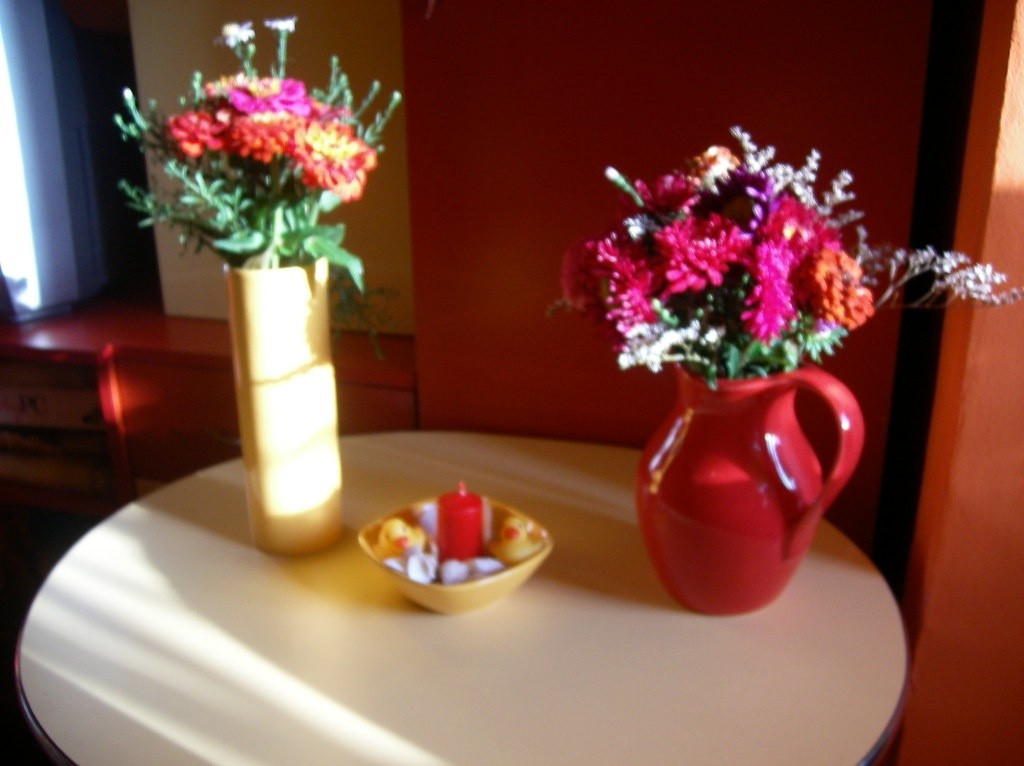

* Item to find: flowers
[113,15,403,362]
[545,124,1024,392]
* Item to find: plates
[358,486,555,614]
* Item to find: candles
[437,480,484,562]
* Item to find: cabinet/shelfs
[0,297,418,521]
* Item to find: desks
[14,429,914,766]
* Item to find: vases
[634,363,865,616]
[222,257,345,558]
